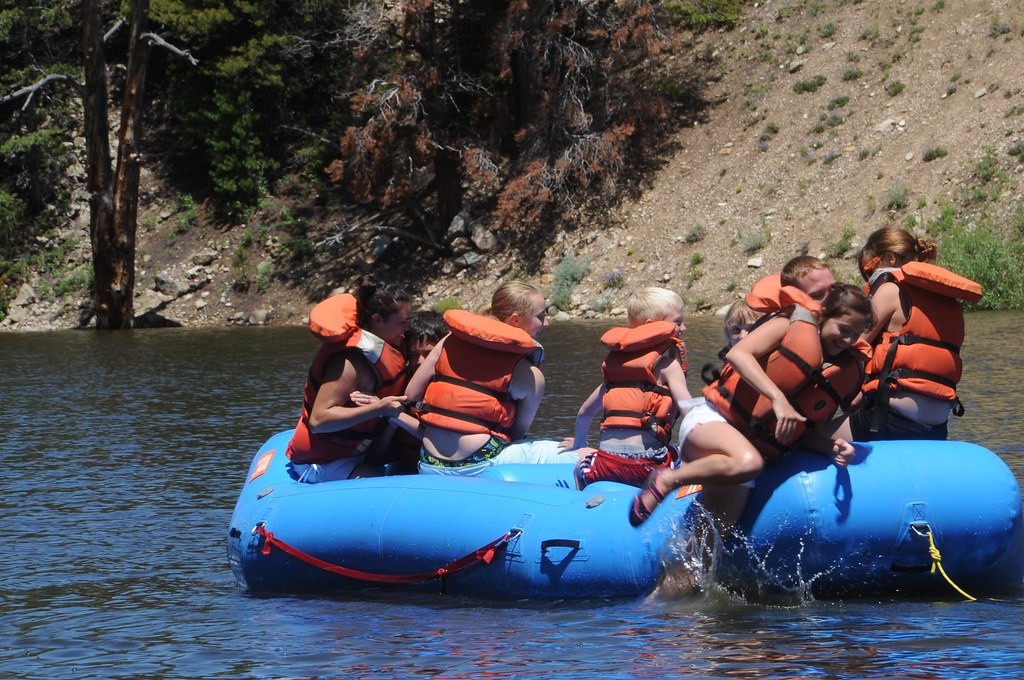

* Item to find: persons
[779,255,837,313]
[722,296,768,347]
[557,287,694,492]
[403,279,597,477]
[286,279,413,485]
[815,225,966,442]
[349,307,449,475]
[628,280,878,603]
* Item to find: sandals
[630,464,670,530]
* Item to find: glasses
[862,253,884,282]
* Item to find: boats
[228,427,1024,603]
